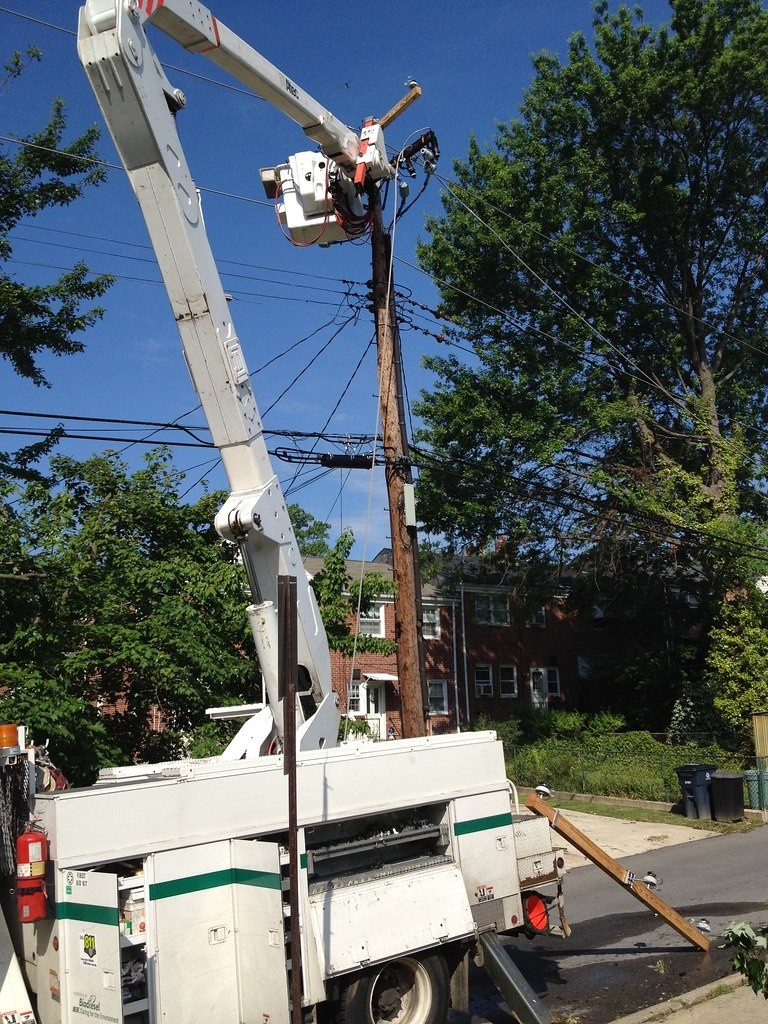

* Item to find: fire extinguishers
[16,819,47,923]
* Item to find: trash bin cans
[745,769,768,810]
[673,764,715,820]
[711,772,744,823]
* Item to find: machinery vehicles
[1,0,573,1024]
